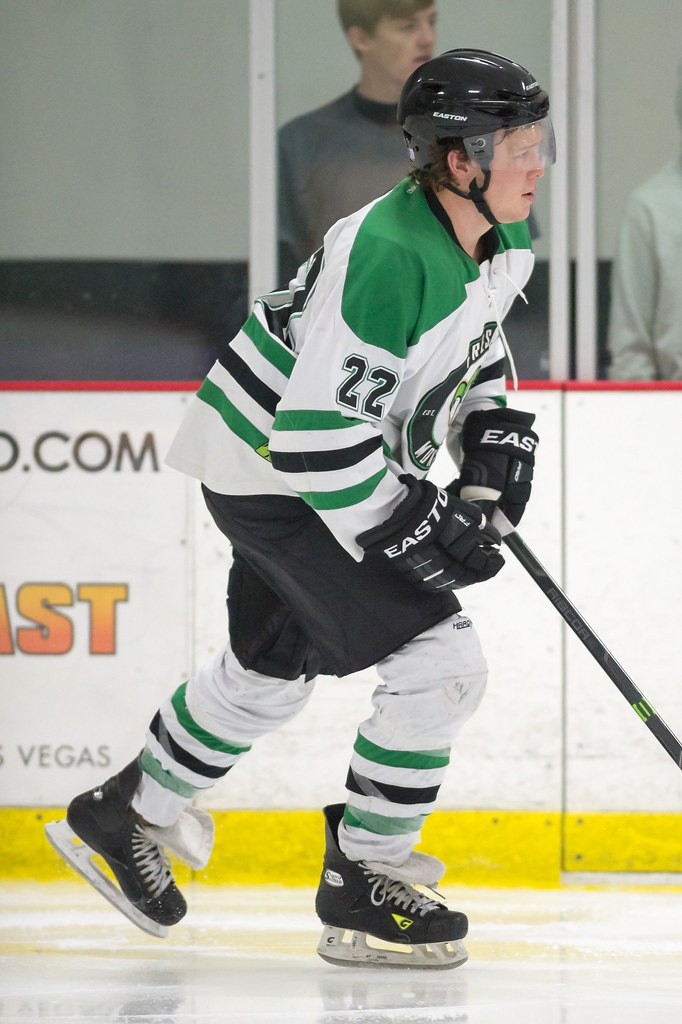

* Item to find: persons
[604,148,682,379]
[277,0,437,291]
[47,48,556,971]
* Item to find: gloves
[457,408,539,528]
[355,473,506,594]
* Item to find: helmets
[397,48,550,175]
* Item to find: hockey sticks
[489,500,682,775]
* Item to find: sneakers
[315,803,469,966]
[44,747,187,938]
